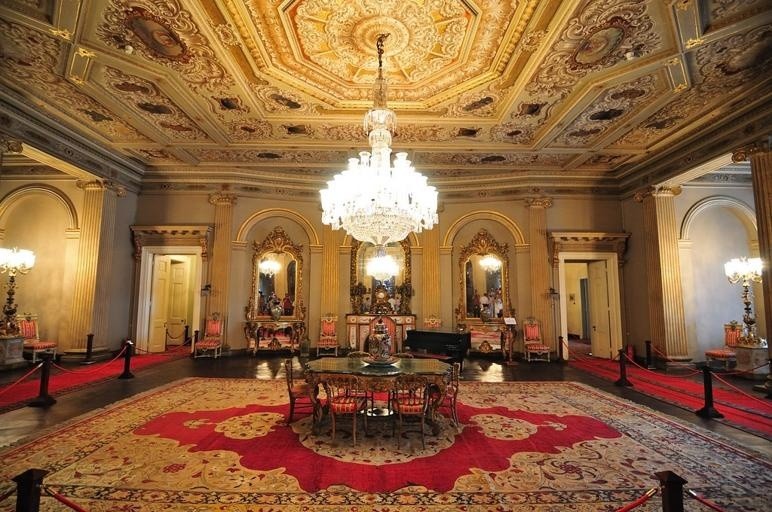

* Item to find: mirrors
[248,225,304,320]
[455,228,511,321]
[350,235,413,315]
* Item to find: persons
[472,287,503,318]
[358,282,405,314]
[258,290,294,315]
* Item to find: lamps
[0,247,34,335]
[318,33,440,256]
[724,256,764,344]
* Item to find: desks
[306,357,449,427]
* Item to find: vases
[272,301,282,321]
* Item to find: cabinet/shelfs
[456,318,516,363]
[345,314,417,355]
[243,320,306,355]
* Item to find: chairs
[389,375,431,451]
[521,317,552,363]
[193,313,225,358]
[16,312,57,363]
[319,375,368,447]
[428,363,460,428]
[316,319,340,358]
[704,320,745,370]
[284,359,319,424]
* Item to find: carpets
[0,374,772,512]
